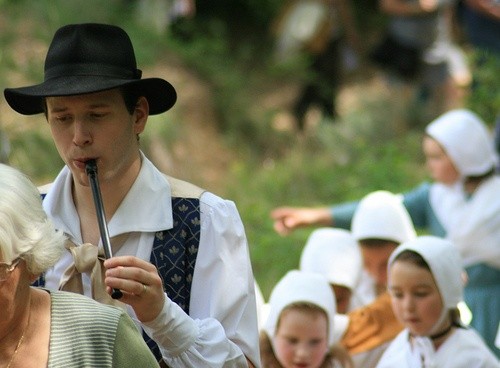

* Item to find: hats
[265,269,337,346]
[299,227,363,298]
[351,191,418,244]
[388,235,463,309]
[425,108,498,177]
[3,23,178,116]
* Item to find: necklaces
[7,291,32,368]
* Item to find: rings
[136,285,146,297]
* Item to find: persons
[299,189,418,368]
[3,23,261,368]
[373,234,499,368]
[259,269,356,368]
[268,108,499,357]
[0,162,160,368]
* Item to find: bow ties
[57,233,128,308]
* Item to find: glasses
[0,258,22,283]
